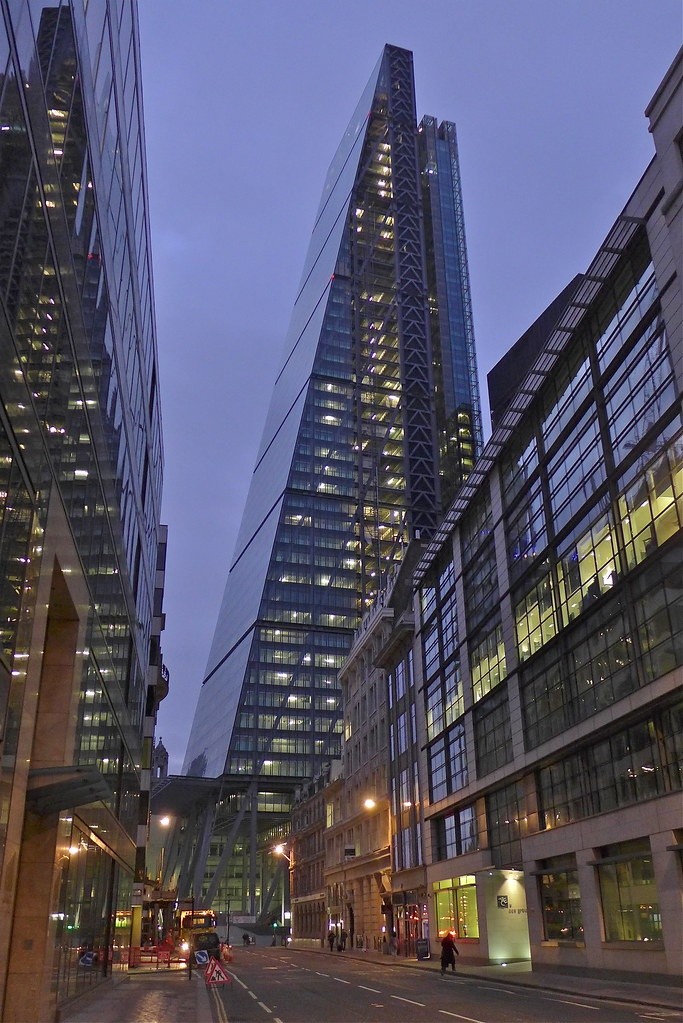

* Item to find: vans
[192,932,221,965]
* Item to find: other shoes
[441,970,444,975]
[452,969,456,972]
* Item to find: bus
[180,910,217,947]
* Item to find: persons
[328,931,335,952]
[440,933,459,975]
[341,929,348,949]
[165,928,175,952]
[284,935,288,947]
[243,932,249,946]
[389,934,398,959]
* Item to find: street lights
[364,799,395,873]
